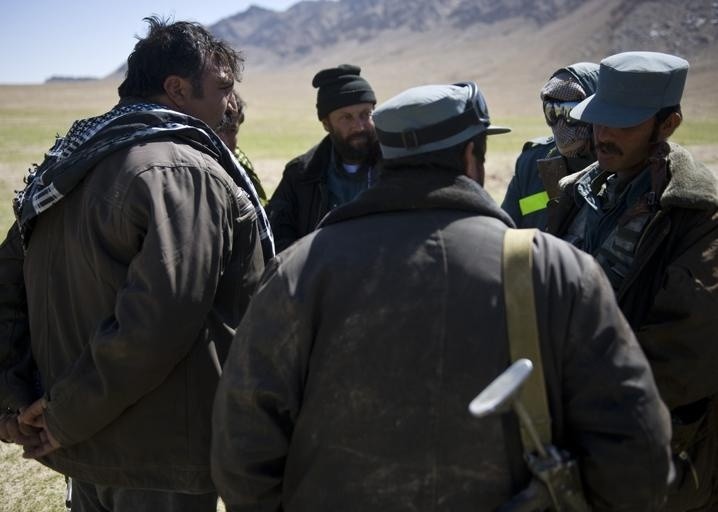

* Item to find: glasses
[543,100,583,126]
[375,82,490,148]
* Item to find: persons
[548,52,716,512]
[497,60,598,248]
[210,78,680,512]
[2,15,255,512]
[260,60,381,261]
[212,91,277,327]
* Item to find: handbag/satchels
[514,450,594,508]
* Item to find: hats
[312,64,376,120]
[373,85,511,158]
[569,51,689,128]
